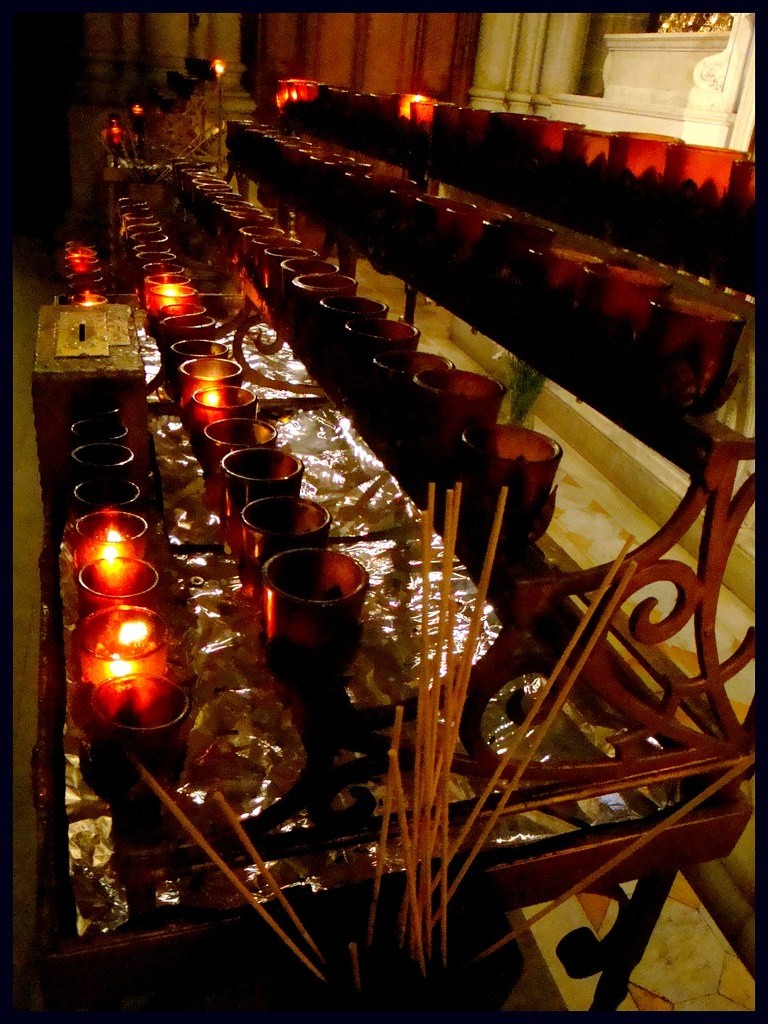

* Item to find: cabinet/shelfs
[38,113,756,1011]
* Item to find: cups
[65,75,755,771]
